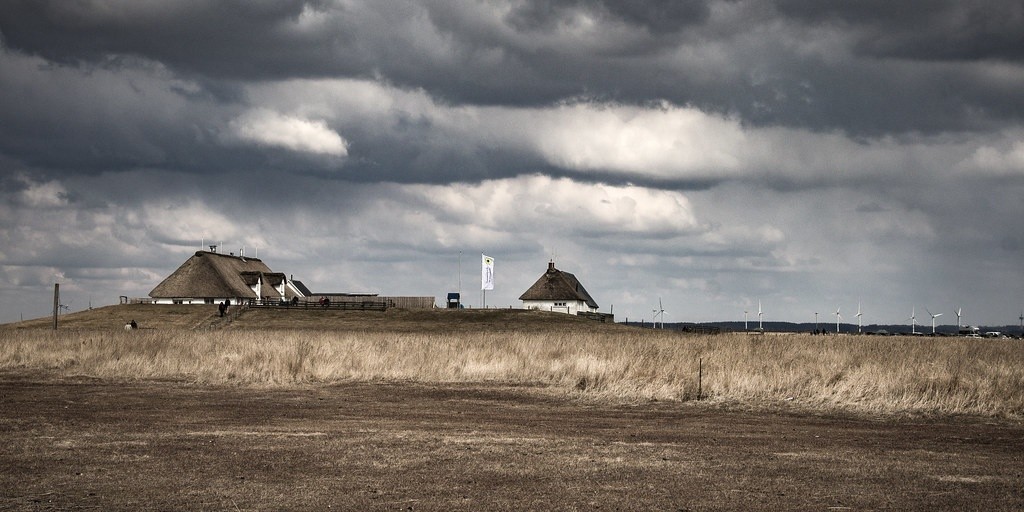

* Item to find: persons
[319,296,330,306]
[224,297,230,314]
[131,320,138,331]
[219,302,225,317]
[124,322,132,332]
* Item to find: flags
[481,254,495,290]
